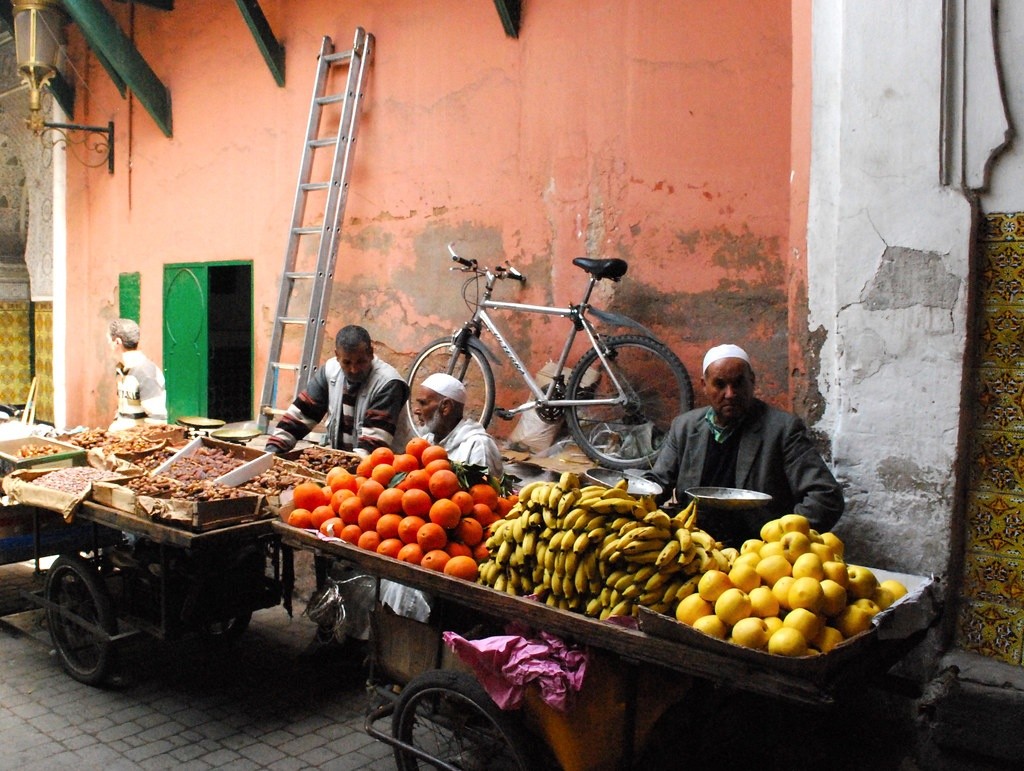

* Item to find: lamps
[13,1,114,174]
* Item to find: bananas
[476,470,738,619]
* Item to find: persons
[642,343,844,553]
[103,318,168,441]
[351,371,504,642]
[261,325,410,645]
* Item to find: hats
[421,372,468,404]
[703,344,751,376]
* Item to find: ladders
[257,29,375,421]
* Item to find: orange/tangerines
[289,439,518,578]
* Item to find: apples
[678,514,907,657]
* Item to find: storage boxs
[0,436,364,534]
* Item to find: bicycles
[403,240,694,472]
